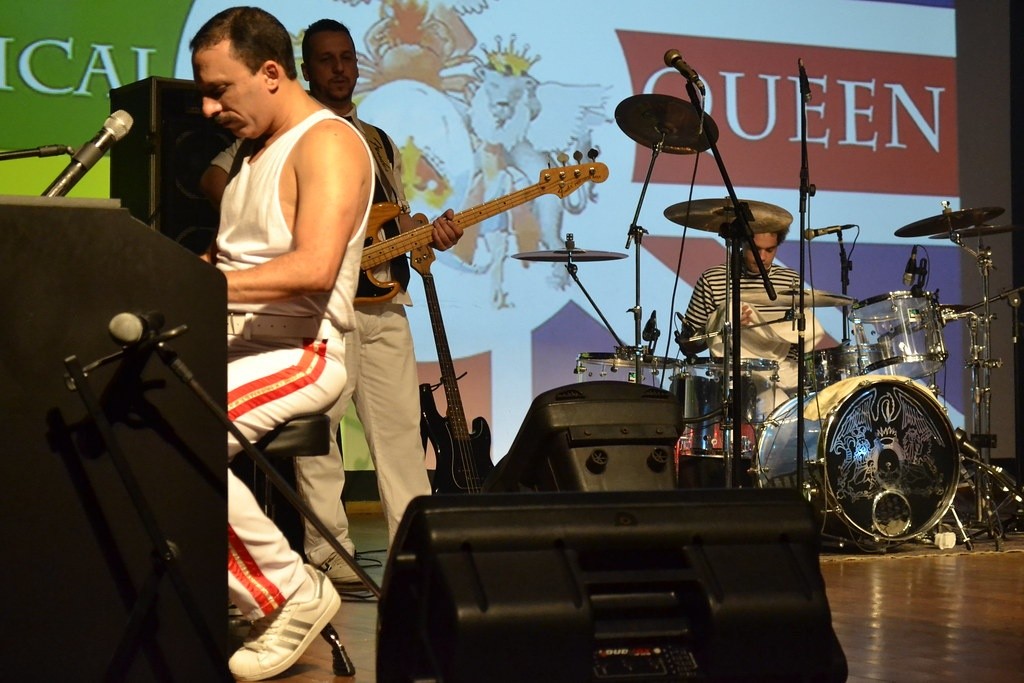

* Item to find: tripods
[943,215,1024,540]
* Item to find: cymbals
[893,206,1008,239]
[928,224,1022,240]
[738,287,855,308]
[938,303,972,312]
[577,349,681,369]
[614,93,720,155]
[663,198,794,234]
[510,249,630,263]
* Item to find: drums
[804,342,896,392]
[669,353,780,460]
[847,290,949,380]
[753,378,962,543]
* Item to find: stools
[230,414,355,678]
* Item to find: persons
[191,6,375,682]
[296,18,464,599]
[681,225,841,435]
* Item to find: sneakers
[228,564,341,681]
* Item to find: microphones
[41,110,133,198]
[106,310,167,346]
[903,245,918,286]
[877,321,911,342]
[663,48,704,89]
[676,312,696,334]
[804,225,856,240]
[643,310,657,341]
[798,58,813,102]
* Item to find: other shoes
[323,558,364,582]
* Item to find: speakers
[111,76,240,264]
[493,379,683,493]
[375,485,849,683]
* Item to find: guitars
[408,211,496,497]
[352,147,611,307]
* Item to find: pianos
[1,193,231,682]
